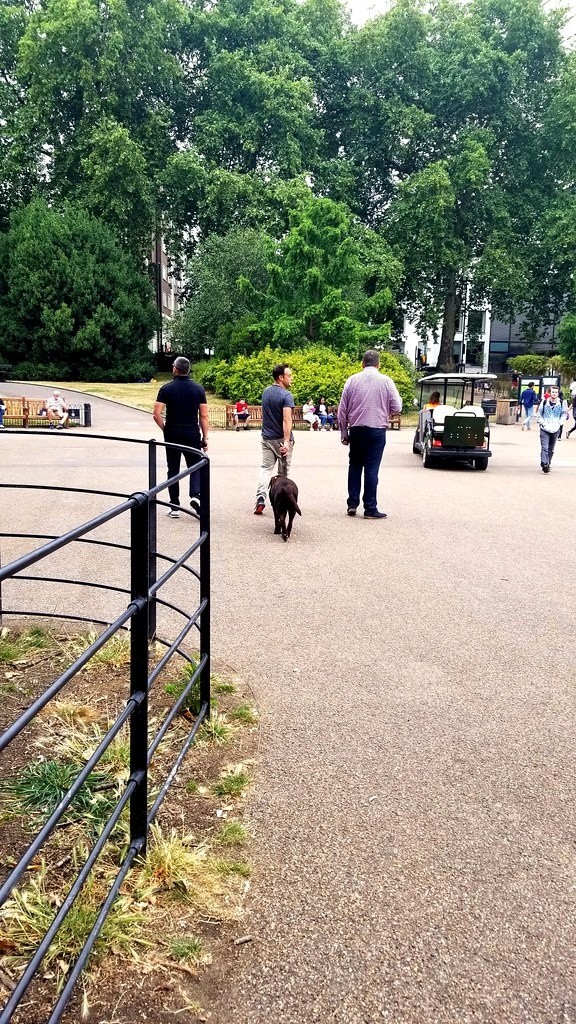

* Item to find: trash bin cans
[496,399,518,425]
[518,372,522,378]
[481,399,497,419]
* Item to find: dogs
[267,475,302,543]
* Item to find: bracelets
[202,437,208,440]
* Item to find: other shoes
[50,424,53,429]
[243,425,250,430]
[542,465,552,474]
[558,438,561,441]
[566,431,570,439]
[521,422,524,431]
[56,424,63,429]
[527,426,531,430]
[346,505,357,516]
[364,510,388,519]
[236,427,240,431]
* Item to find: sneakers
[189,497,201,517]
[253,500,265,515]
[165,509,181,518]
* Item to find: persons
[475,372,492,395]
[45,391,68,429]
[517,382,540,431]
[314,398,333,431]
[234,397,251,431]
[154,358,209,518]
[424,391,441,411]
[537,386,566,474]
[567,396,576,438]
[542,387,568,441]
[570,377,576,399]
[337,350,403,518]
[0,398,7,428]
[254,364,295,514]
[303,398,319,431]
[512,370,519,390]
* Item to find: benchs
[0,397,69,428]
[225,404,401,432]
[432,405,489,436]
